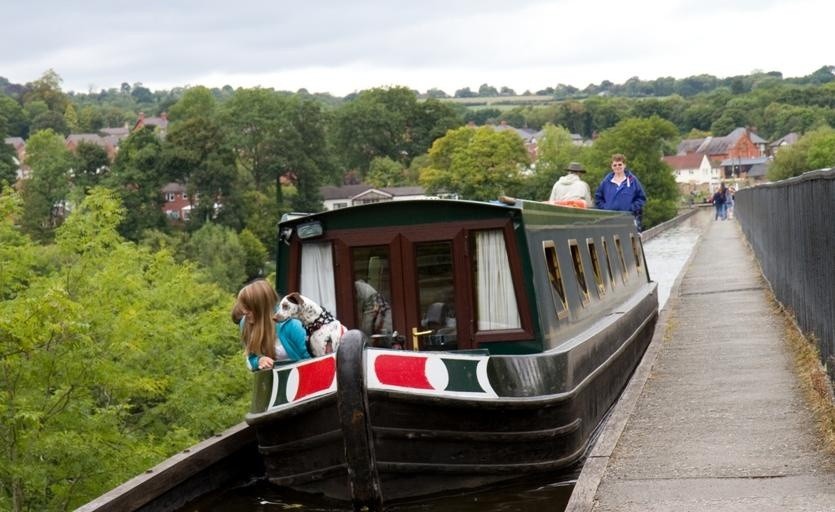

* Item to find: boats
[240,194,658,507]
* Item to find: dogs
[272,292,349,358]
[354,280,393,335]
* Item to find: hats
[562,161,587,173]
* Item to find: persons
[426,293,452,345]
[237,279,312,370]
[232,304,243,324]
[550,163,591,207]
[594,154,646,232]
[712,187,736,221]
